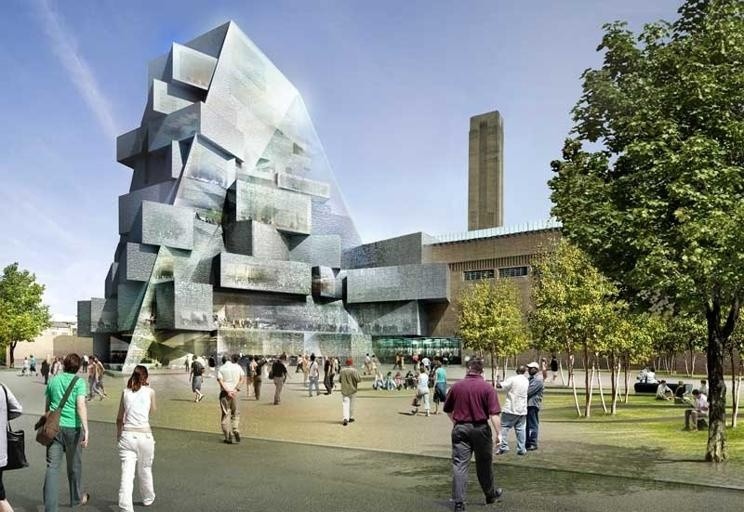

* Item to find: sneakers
[73,492,89,508]
[223,429,240,444]
[343,419,347,425]
[349,418,354,422]
[193,394,204,403]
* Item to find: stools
[674,397,681,403]
[697,417,708,431]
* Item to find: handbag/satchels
[0,429,30,471]
[34,411,61,446]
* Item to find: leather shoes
[454,502,465,512]
[486,487,503,504]
[526,443,538,450]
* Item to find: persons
[42,353,90,511]
[0,382,23,511]
[635,364,709,431]
[114,366,156,511]
[441,357,502,512]
[17,354,109,402]
[182,349,560,456]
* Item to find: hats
[346,356,353,366]
[526,362,539,370]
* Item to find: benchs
[635,383,693,393]
